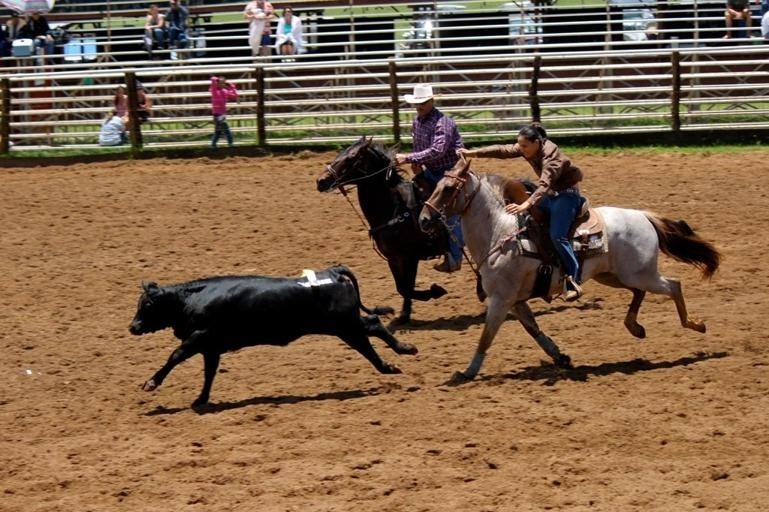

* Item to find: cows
[128,266,419,412]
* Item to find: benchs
[0,1,768,140]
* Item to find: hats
[403,83,443,105]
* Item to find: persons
[760,10,769,39]
[164,0,189,49]
[456,122,583,301]
[722,0,755,39]
[113,87,130,126]
[28,12,56,65]
[243,0,274,62]
[135,87,150,122]
[144,4,166,50]
[274,6,301,63]
[208,75,237,147]
[394,84,465,272]
[0,12,30,66]
[98,109,128,146]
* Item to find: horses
[316,135,540,328]
[418,153,725,383]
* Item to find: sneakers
[433,254,462,271]
[565,289,578,302]
